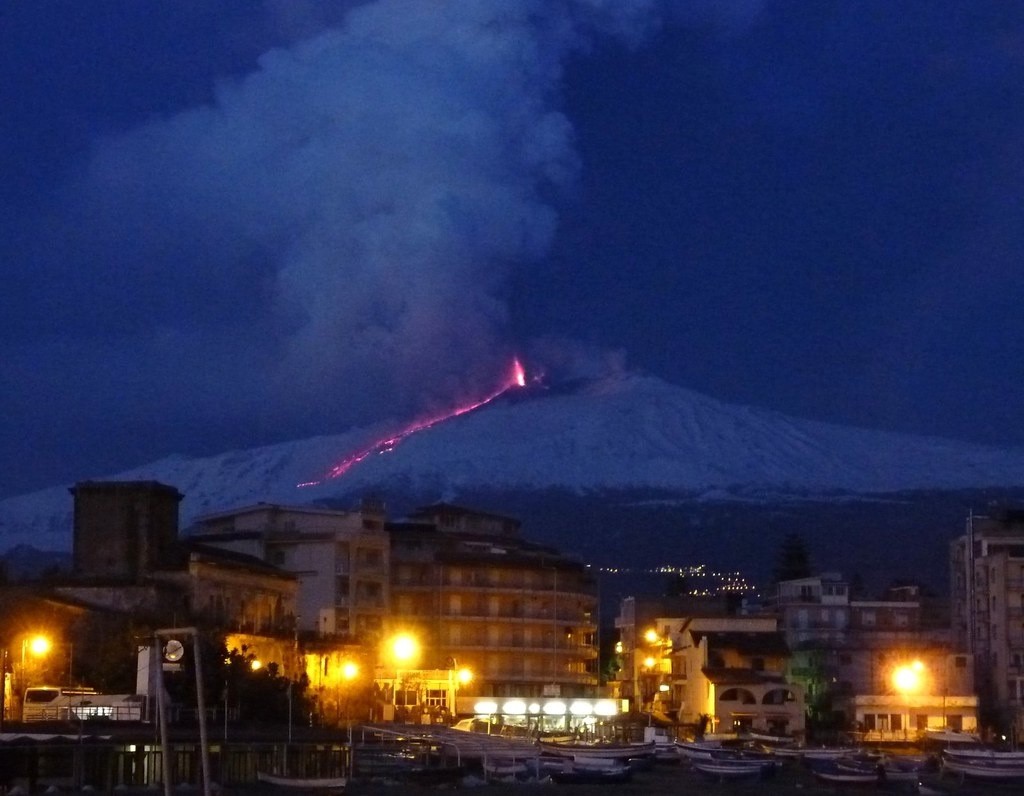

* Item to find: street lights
[21,638,46,704]
[450,671,470,717]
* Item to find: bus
[23,687,100,724]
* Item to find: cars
[452,717,481,734]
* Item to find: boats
[473,726,1023,779]
